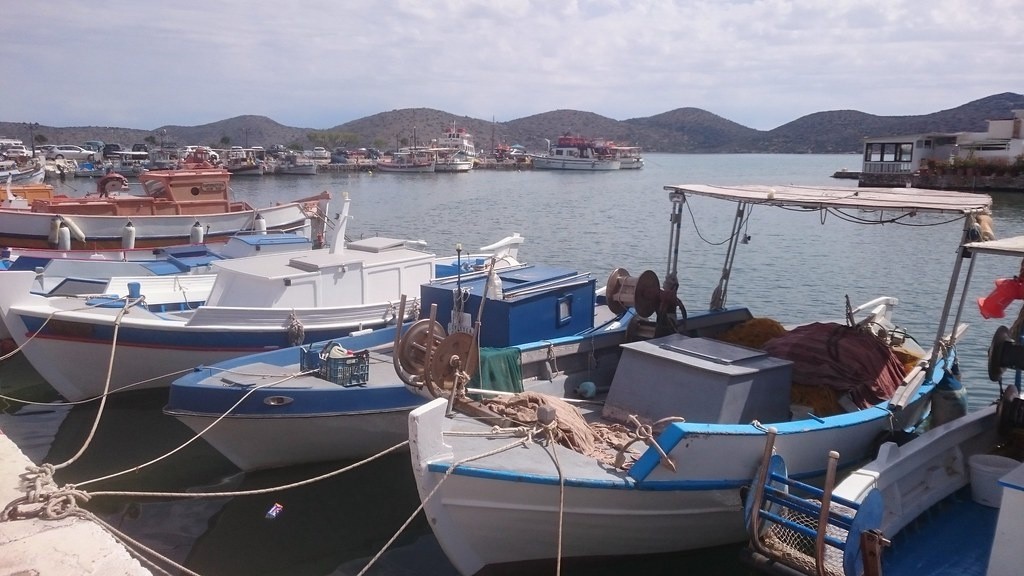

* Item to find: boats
[432,122,473,170]
[739,238,1024,576]
[166,244,679,474]
[0,160,524,406]
[531,135,620,170]
[621,147,644,168]
[377,147,434,172]
[412,180,994,576]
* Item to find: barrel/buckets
[966,453,1023,509]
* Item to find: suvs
[182,146,219,161]
[46,145,96,161]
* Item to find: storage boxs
[299,342,370,387]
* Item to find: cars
[330,147,349,162]
[35,145,54,154]
[103,143,126,158]
[230,146,246,160]
[84,141,104,159]
[7,145,27,157]
[133,144,148,159]
[312,147,327,158]
[358,148,366,152]
